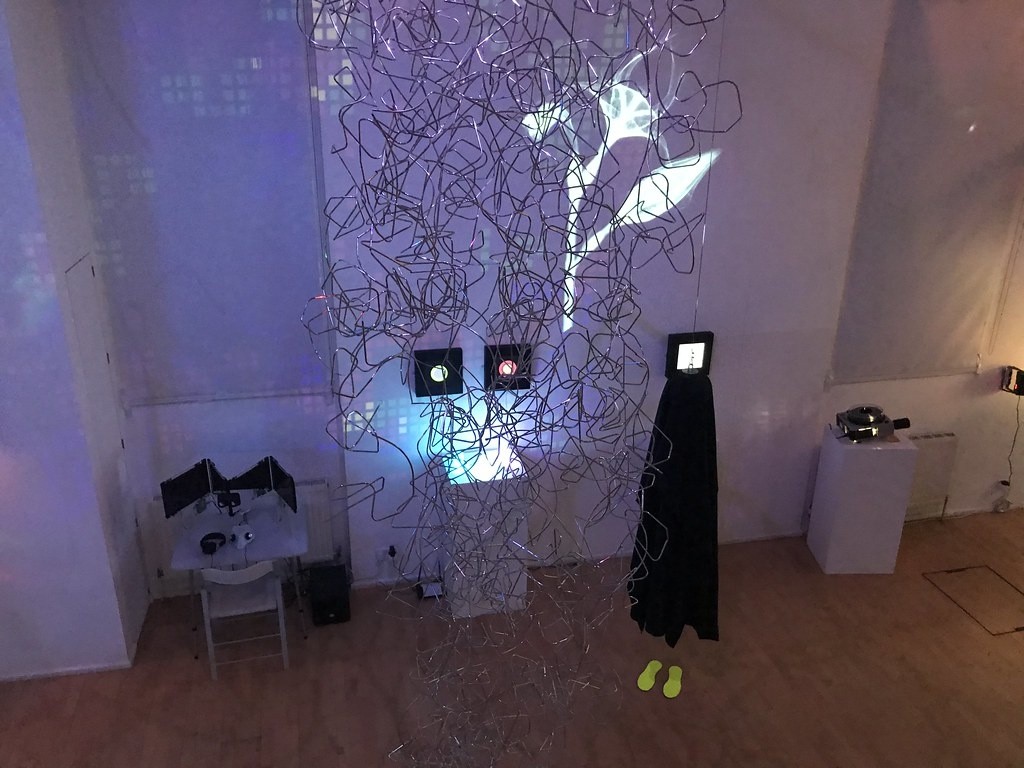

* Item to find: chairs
[201,561,290,679]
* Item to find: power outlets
[374,548,396,561]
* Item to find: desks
[170,490,310,660]
[808,427,918,575]
